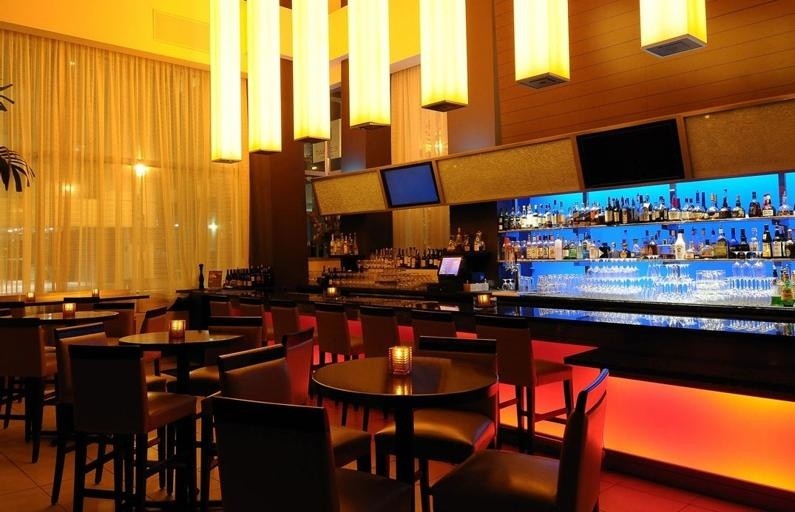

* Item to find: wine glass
[395,268,437,290]
[502,278,515,293]
[727,251,775,307]
[356,260,394,279]
[333,273,377,289]
[517,263,725,305]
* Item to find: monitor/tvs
[574,118,686,190]
[379,161,442,209]
[438,255,464,278]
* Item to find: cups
[537,308,773,334]
[169,318,187,340]
[388,345,413,376]
[27,289,99,317]
[387,377,413,395]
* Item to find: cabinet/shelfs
[497,169,794,337]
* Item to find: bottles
[329,231,359,256]
[224,262,273,289]
[446,226,471,253]
[396,246,444,269]
[472,230,486,252]
[770,267,795,307]
[321,266,344,286]
[774,323,795,336]
[373,248,393,260]
[496,190,795,262]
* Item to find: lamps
[208,0,242,163]
[294,0,330,145]
[514,2,569,92]
[348,2,392,129]
[639,1,707,58]
[247,0,281,156]
[421,1,467,113]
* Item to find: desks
[309,355,499,488]
[1,287,150,312]
[21,310,120,346]
[117,331,243,395]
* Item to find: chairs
[219,343,372,475]
[92,301,136,338]
[412,308,458,353]
[198,325,315,512]
[209,294,232,315]
[52,320,167,506]
[269,300,302,346]
[372,334,498,512]
[239,295,274,347]
[359,304,402,432]
[429,367,610,511]
[315,301,365,427]
[188,316,263,448]
[1,301,58,404]
[476,313,577,455]
[139,306,167,377]
[0,316,58,464]
[66,343,198,512]
[206,394,417,511]
